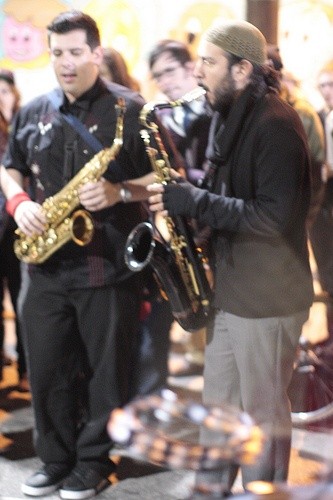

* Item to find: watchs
[119,181,132,204]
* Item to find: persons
[148,19,314,500]
[149,39,218,377]
[263,44,333,351]
[1,70,33,414]
[98,47,171,414]
[0,9,171,500]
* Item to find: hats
[207,19,268,65]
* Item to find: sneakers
[60,468,108,500]
[22,466,62,496]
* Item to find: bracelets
[5,193,30,215]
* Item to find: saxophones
[126,93,214,332]
[13,96,136,262]
